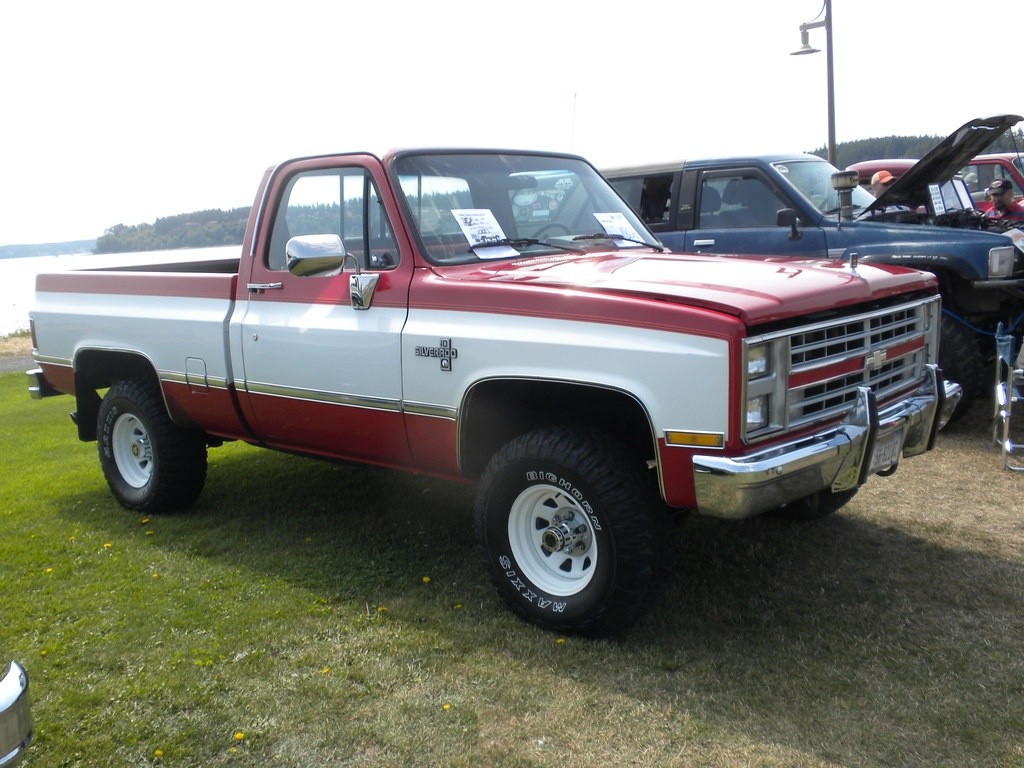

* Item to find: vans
[960,151,1024,221]
[846,157,975,230]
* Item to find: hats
[989,179,1013,195]
[871,170,898,185]
[965,173,978,184]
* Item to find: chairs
[991,321,1024,471]
[640,176,778,227]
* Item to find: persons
[870,170,899,199]
[963,172,980,193]
[984,178,1024,227]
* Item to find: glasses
[878,180,894,186]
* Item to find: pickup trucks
[487,113,1023,429]
[24,144,959,640]
